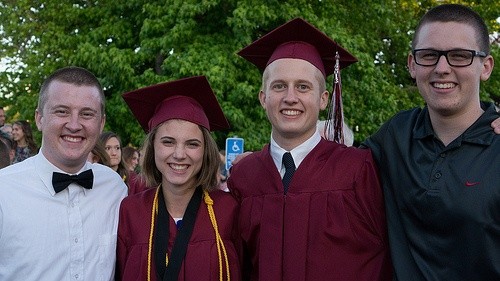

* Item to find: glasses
[411,48,487,67]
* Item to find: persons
[0,106,39,170]
[226,18,392,281]
[0,66,129,281]
[232,4,500,281]
[86,75,240,281]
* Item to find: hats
[235,17,358,145]
[121,75,231,136]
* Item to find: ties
[282,152,296,195]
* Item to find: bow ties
[52,169,93,193]
